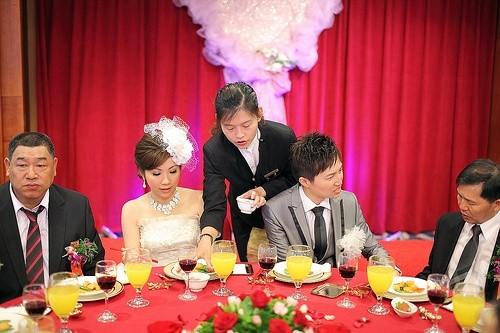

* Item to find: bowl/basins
[237,197,259,215]
[184,273,210,292]
[391,299,418,318]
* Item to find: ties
[448,224,482,297]
[311,206,327,262]
[19,205,45,284]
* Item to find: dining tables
[0,262,466,333]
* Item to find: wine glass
[95,260,118,323]
[176,245,199,301]
[367,255,395,316]
[123,249,152,308]
[336,240,359,308]
[452,282,485,333]
[257,244,277,296]
[286,244,313,302]
[425,274,450,333]
[211,240,237,296]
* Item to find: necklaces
[150,189,180,215]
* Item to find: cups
[49,272,80,333]
[18,315,54,333]
[23,287,47,317]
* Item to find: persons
[120,114,220,270]
[260,128,403,282]
[413,157,500,313]
[0,131,106,308]
[195,81,305,268]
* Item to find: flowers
[262,47,297,72]
[62,237,98,267]
[485,244,500,284]
[148,287,351,333]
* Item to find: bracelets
[197,234,213,247]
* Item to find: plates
[269,259,331,284]
[61,277,123,302]
[163,252,219,281]
[382,276,440,302]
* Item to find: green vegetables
[80,285,88,291]
[198,265,210,273]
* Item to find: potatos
[83,281,100,290]
[393,280,418,292]
[194,262,203,271]
